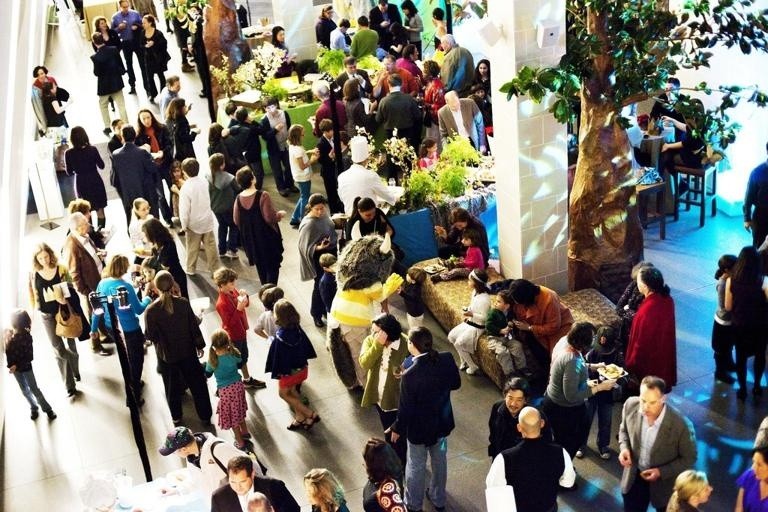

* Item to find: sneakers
[243,377,266,388]
[224,249,239,258]
[576,445,584,458]
[459,361,467,370]
[466,365,479,375]
[234,441,248,454]
[32,405,39,420]
[448,261,455,271]
[240,433,251,440]
[291,219,301,225]
[184,267,195,275]
[47,411,57,419]
[599,445,611,460]
[314,314,323,327]
[431,274,443,282]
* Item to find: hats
[158,425,194,456]
[350,134,370,163]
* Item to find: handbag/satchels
[221,139,255,174]
[339,221,349,253]
[55,299,83,338]
[422,104,432,128]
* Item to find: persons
[6,198,462,512]
[636,77,712,219]
[448,141,768,512]
[18,0,492,288]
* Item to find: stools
[672,161,719,227]
[632,181,667,240]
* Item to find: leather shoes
[713,362,767,402]
[127,399,145,408]
[279,188,288,197]
[94,348,112,356]
[286,186,299,192]
[182,48,196,73]
[129,88,158,102]
[68,389,76,397]
[426,487,445,512]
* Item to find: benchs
[406,255,623,391]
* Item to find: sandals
[287,419,306,431]
[303,411,321,431]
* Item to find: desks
[82,0,121,42]
[625,115,677,216]
[215,84,322,176]
[241,24,273,50]
[385,179,497,279]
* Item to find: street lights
[89,285,153,483]
